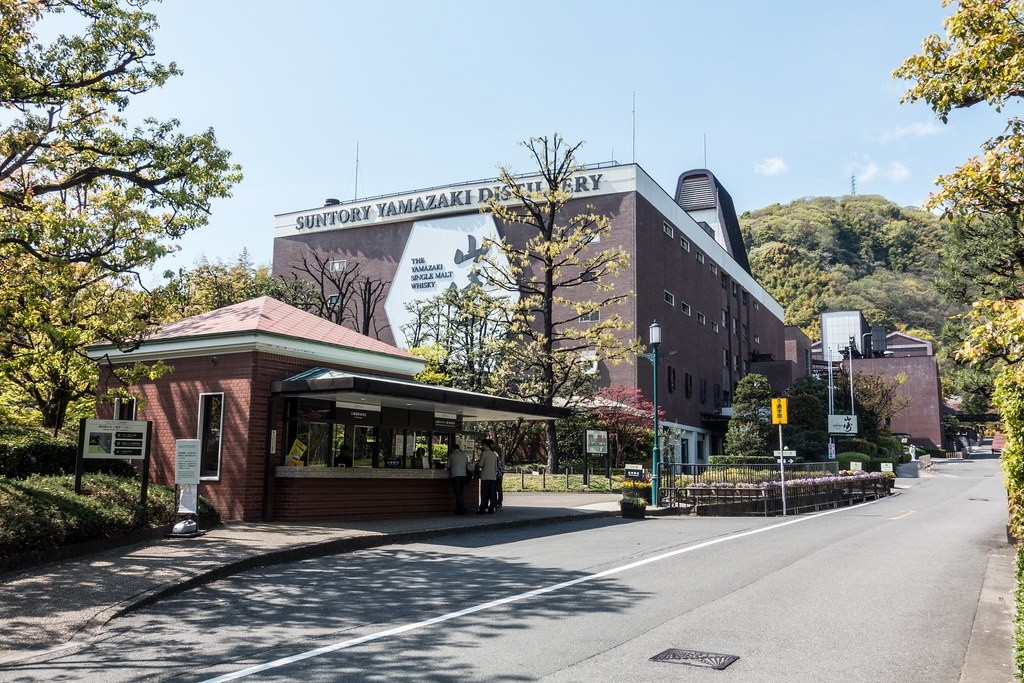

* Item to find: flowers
[690,471,886,489]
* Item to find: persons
[445,443,468,514]
[413,446,435,469]
[334,444,353,467]
[475,438,507,515]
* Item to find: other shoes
[486,510,494,514]
[476,510,486,515]
[496,504,502,511]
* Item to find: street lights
[648,319,661,505]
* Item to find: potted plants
[885,471,896,488]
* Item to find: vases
[688,480,874,496]
